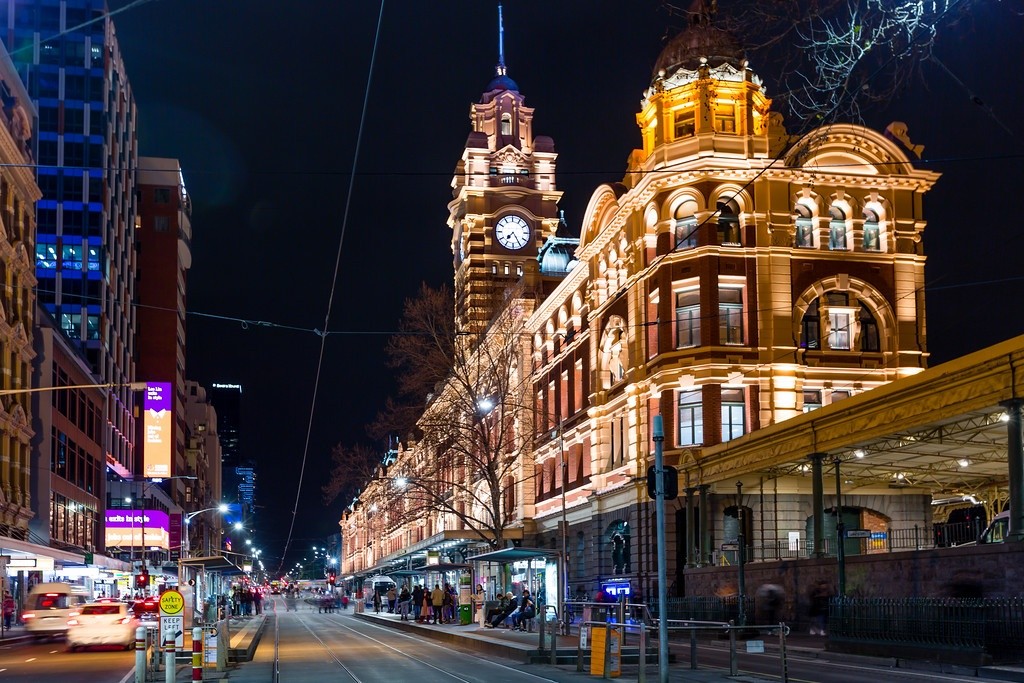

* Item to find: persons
[470,584,487,630]
[341,593,349,609]
[233,588,262,615]
[595,587,643,623]
[485,589,541,631]
[388,583,459,625]
[96,591,150,600]
[371,588,382,614]
[1,588,15,632]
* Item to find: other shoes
[810,630,816,634]
[432,622,436,624]
[820,631,826,635]
[401,617,404,620]
[405,617,408,621]
[485,624,493,628]
[445,620,451,624]
[512,626,520,629]
[439,621,443,624]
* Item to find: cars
[67,596,161,652]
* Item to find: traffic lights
[135,575,148,584]
[330,576,335,585]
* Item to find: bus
[362,576,397,606]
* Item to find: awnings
[463,546,561,561]
[415,562,473,572]
[386,570,422,577]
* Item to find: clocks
[495,214,531,251]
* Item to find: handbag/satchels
[524,604,531,612]
[475,603,482,609]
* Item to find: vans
[23,580,92,644]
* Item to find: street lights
[125,498,134,600]
[481,401,566,637]
[186,505,228,580]
[142,476,197,574]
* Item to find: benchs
[505,614,534,633]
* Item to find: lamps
[958,459,973,467]
[823,382,834,391]
[853,383,864,392]
